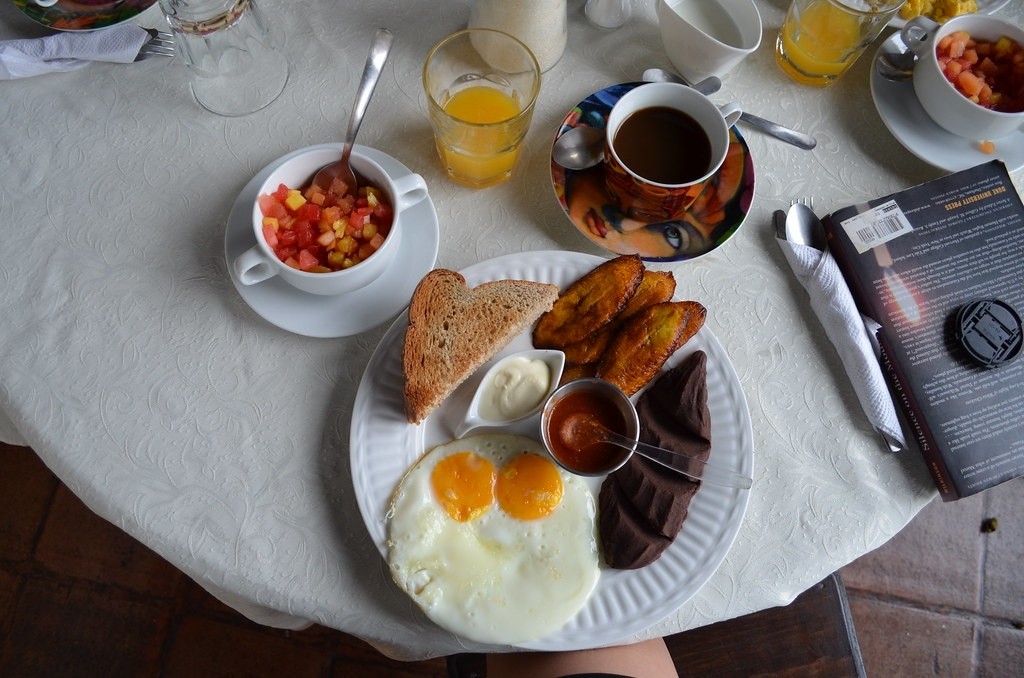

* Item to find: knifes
[771,210,789,240]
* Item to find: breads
[403,268,558,426]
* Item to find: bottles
[584,0,633,30]
[468,0,568,74]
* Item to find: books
[825,158,1024,502]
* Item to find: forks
[138,31,174,56]
[789,197,814,213]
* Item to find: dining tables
[1,0,1024,664]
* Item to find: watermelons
[258,184,391,270]
[935,28,1024,114]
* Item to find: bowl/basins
[541,379,641,478]
[455,349,565,440]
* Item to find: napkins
[775,233,910,451]
[0,23,153,86]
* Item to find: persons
[487,637,679,678]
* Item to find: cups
[421,27,541,190]
[900,15,1023,140]
[159,0,289,117]
[604,80,743,223]
[654,0,762,85]
[773,0,908,92]
[233,148,428,296]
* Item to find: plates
[830,0,1009,31]
[869,29,1024,175]
[224,142,440,339]
[348,251,753,651]
[11,0,159,32]
[550,81,756,262]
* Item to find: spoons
[785,203,903,452]
[642,68,817,150]
[141,29,158,46]
[552,77,722,170]
[875,33,928,84]
[559,413,753,490]
[311,28,394,198]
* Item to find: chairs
[448,570,865,677]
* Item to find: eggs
[383,434,600,644]
